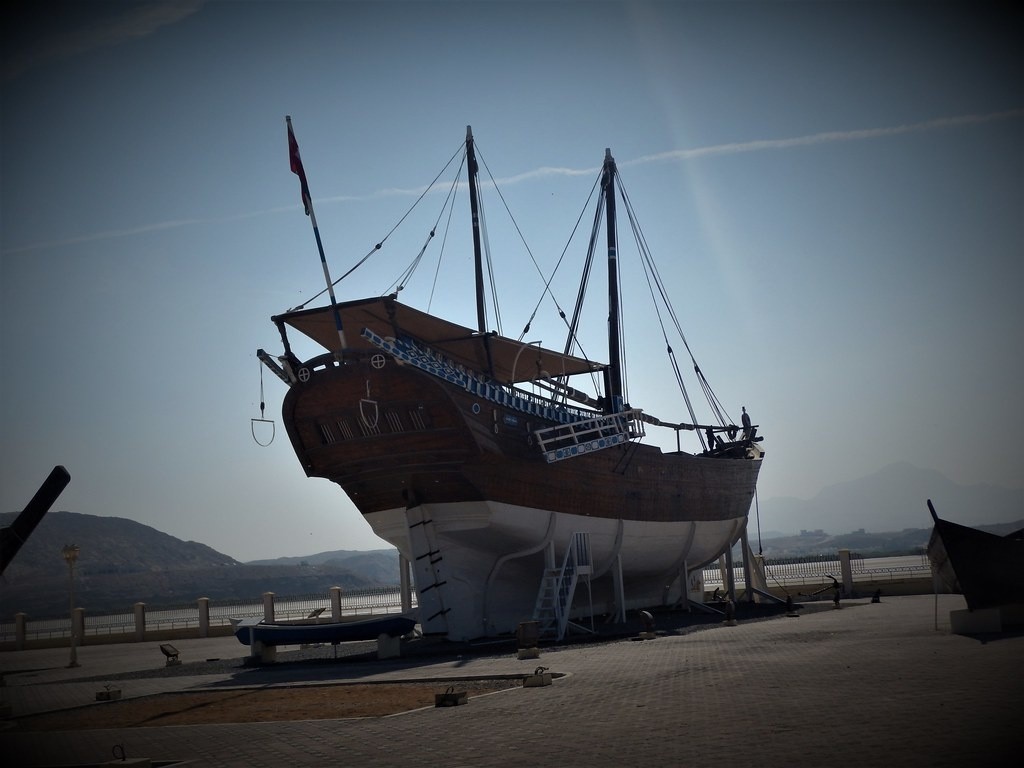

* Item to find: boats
[228,608,419,645]
[926,499,1024,611]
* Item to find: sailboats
[251,116,767,648]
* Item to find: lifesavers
[727,425,737,440]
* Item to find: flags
[287,121,312,216]
